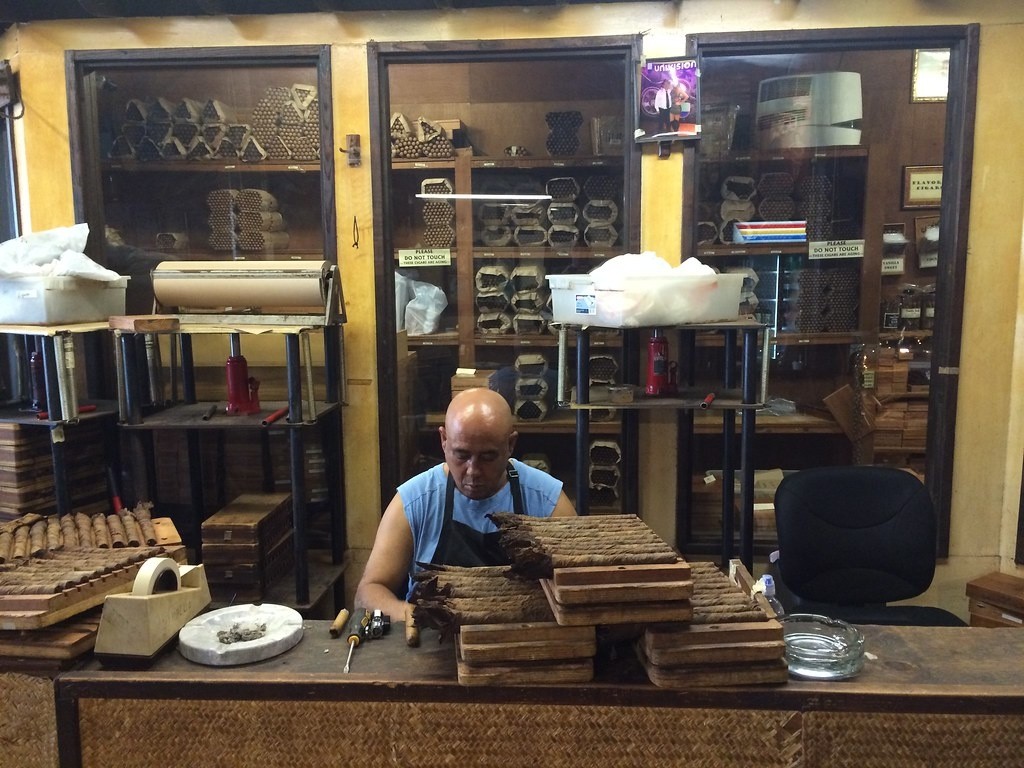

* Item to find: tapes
[131,556,181,597]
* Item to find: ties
[665,91,670,110]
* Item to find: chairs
[774,465,967,628]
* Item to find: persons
[655,76,689,135]
[353,387,578,625]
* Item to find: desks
[55,618,1024,768]
[0,605,100,768]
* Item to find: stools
[966,572,1024,627]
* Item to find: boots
[670,119,680,136]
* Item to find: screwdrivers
[343,606,371,673]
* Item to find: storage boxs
[0,276,132,326]
[545,271,747,329]
[202,491,295,603]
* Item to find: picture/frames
[632,54,700,143]
[901,165,944,209]
[909,46,949,103]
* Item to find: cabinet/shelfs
[0,24,954,619]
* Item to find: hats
[661,79,671,87]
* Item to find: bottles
[760,574,785,618]
